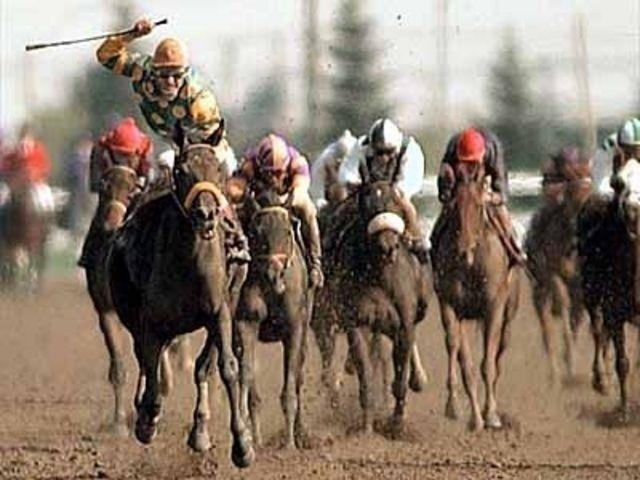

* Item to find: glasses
[154,71,184,77]
[377,148,393,155]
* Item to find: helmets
[108,118,141,153]
[151,38,185,68]
[619,118,640,144]
[256,133,289,170]
[457,130,485,161]
[369,118,398,147]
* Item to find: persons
[97,20,252,265]
[429,128,528,263]
[0,119,175,286]
[216,119,433,287]
[532,117,640,228]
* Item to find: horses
[523,153,596,377]
[232,154,315,450]
[308,165,392,401]
[104,118,254,469]
[79,137,192,438]
[576,174,638,423]
[321,137,429,438]
[430,160,519,433]
